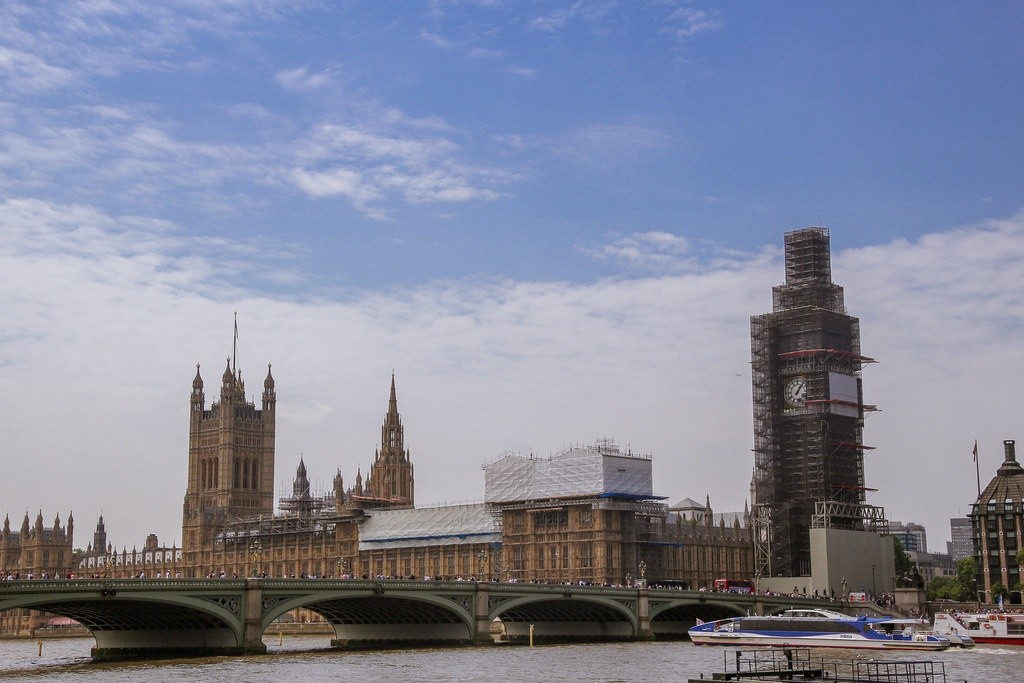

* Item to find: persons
[0,569,1024,621]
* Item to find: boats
[784,605,974,647]
[688,617,949,651]
[933,612,1024,645]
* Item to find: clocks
[783,376,807,408]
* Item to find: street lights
[872,563,875,598]
[103,552,116,579]
[249,542,263,577]
[841,578,847,600]
[625,573,632,587]
[479,550,487,581]
[755,569,760,594]
[639,560,647,589]
[973,578,977,601]
[338,558,347,578]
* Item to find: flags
[972,444,977,462]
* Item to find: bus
[714,579,755,594]
